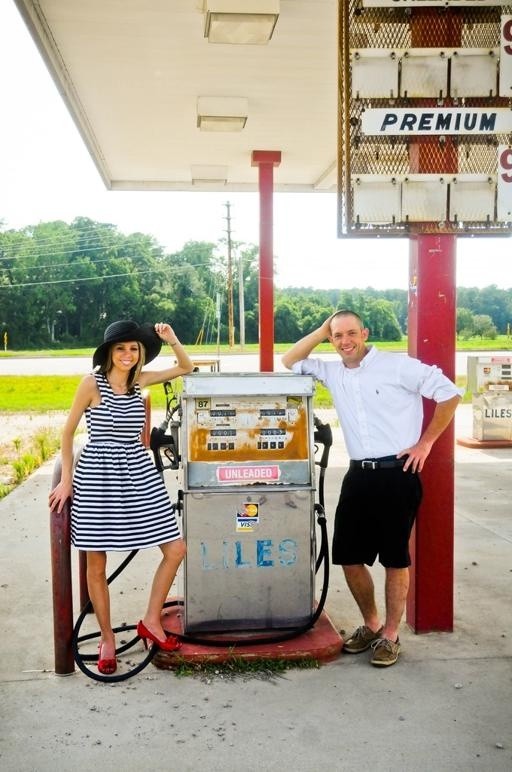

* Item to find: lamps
[188,163,230,187]
[192,95,251,133]
[199,0,284,45]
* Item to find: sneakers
[369,632,402,667]
[343,624,385,654]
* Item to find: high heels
[135,619,184,653]
[97,635,118,675]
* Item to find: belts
[346,453,412,471]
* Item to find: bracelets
[170,339,179,347]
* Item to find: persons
[281,309,464,666]
[48,319,195,675]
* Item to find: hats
[91,318,163,370]
